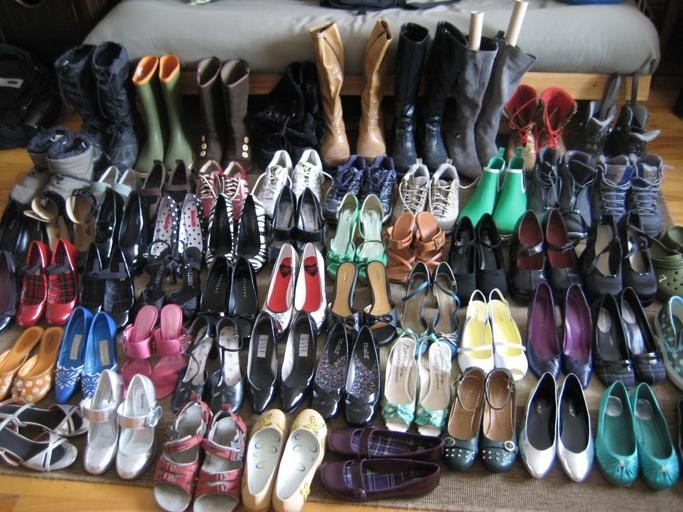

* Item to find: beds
[78,1,662,132]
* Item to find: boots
[198,56,221,174]
[288,67,322,168]
[132,53,168,176]
[157,54,192,176]
[240,61,302,167]
[386,23,431,172]
[10,122,93,208]
[218,56,256,171]
[418,21,465,175]
[584,100,663,158]
[475,35,535,164]
[309,24,351,167]
[356,20,393,167]
[56,45,103,176]
[502,84,579,175]
[445,36,496,180]
[92,37,136,177]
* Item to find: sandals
[151,398,212,512]
[192,404,247,512]
[0,413,76,472]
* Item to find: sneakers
[441,367,489,471]
[480,370,516,470]
[628,384,682,488]
[596,380,637,487]
[518,373,556,483]
[557,374,596,485]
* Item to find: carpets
[0,182,682,509]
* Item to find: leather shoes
[318,456,441,502]
[271,407,329,512]
[116,373,157,479]
[331,428,441,458]
[242,409,285,512]
[79,369,123,474]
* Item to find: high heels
[0,146,683,437]
